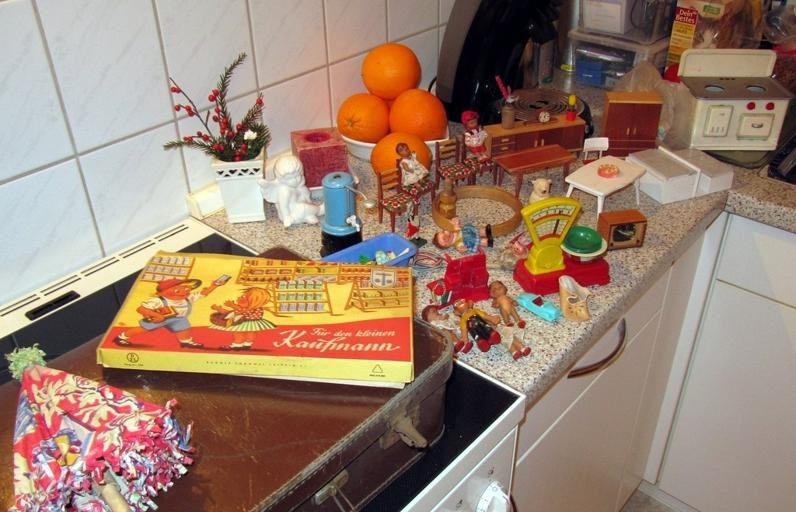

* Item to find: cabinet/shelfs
[510,229,706,512]
[658,213,796,512]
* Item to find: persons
[210,287,280,352]
[112,277,226,349]
[269,154,325,227]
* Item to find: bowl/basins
[344,122,451,167]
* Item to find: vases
[210,145,268,224]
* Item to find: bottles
[556,63,577,95]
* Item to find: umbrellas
[3,345,201,512]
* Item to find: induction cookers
[0,218,525,511]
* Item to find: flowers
[163,51,272,162]
